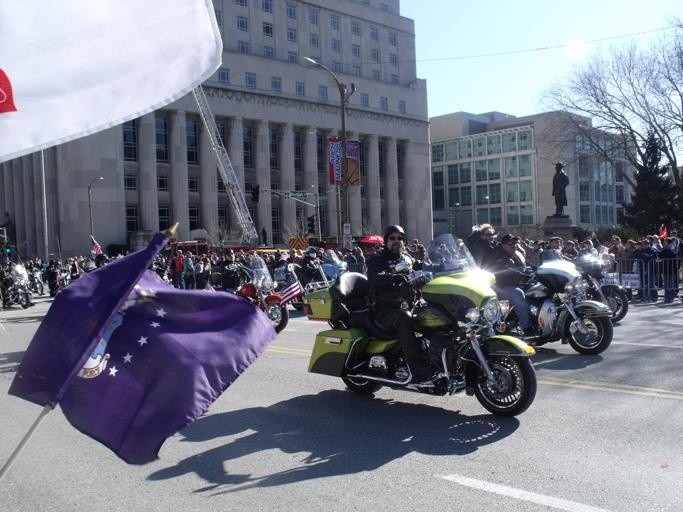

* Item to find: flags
[8,232,278,465]
[279,274,305,308]
[0,0,223,164]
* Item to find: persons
[275,242,385,287]
[366,224,462,382]
[1,255,96,308]
[92,243,277,295]
[609,226,683,303]
[552,163,569,215]
[465,224,610,335]
[402,238,464,270]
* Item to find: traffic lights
[252,184,260,203]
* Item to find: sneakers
[413,364,432,378]
[523,326,543,336]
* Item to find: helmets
[306,248,318,254]
[383,225,405,243]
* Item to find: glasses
[482,233,494,237]
[506,243,515,246]
[390,236,404,240]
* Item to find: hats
[501,234,519,243]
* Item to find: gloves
[387,273,410,288]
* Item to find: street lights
[448,202,460,233]
[88,177,103,250]
[474,195,489,226]
[299,56,358,248]
